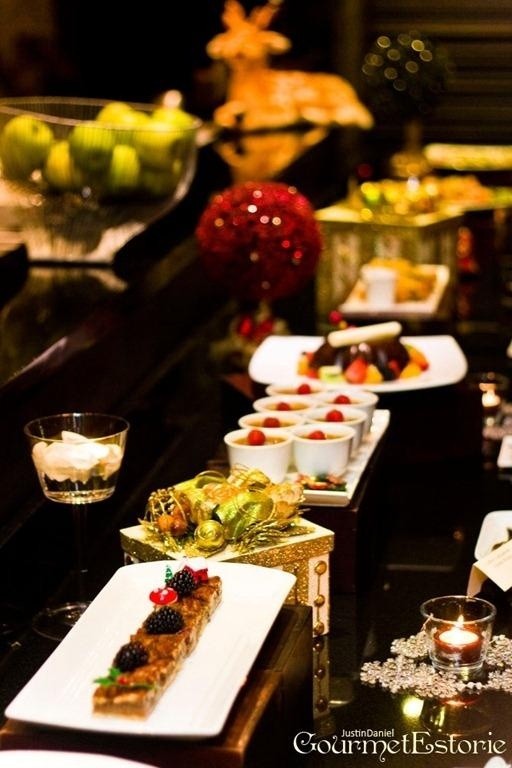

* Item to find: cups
[23,411,130,507]
[419,594,498,676]
[223,383,379,483]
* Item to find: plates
[335,258,451,321]
[226,408,392,509]
[246,334,469,393]
[4,553,299,744]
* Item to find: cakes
[93,565,224,718]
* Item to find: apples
[0,101,198,197]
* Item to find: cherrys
[247,384,350,446]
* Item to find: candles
[432,615,481,664]
